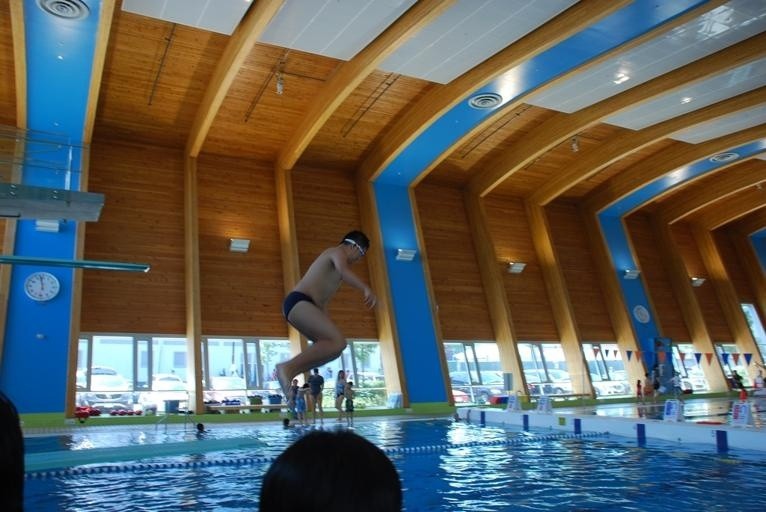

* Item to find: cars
[345,370,386,389]
[75,366,280,412]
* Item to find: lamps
[623,269,641,279]
[691,277,706,287]
[229,238,251,253]
[34,219,59,232]
[506,262,527,274]
[395,248,416,260]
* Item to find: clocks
[23,271,60,301]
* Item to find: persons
[345,369,352,380]
[344,381,355,427]
[528,385,553,395]
[195,423,216,441]
[284,367,333,427]
[727,369,766,390]
[635,365,685,404]
[334,370,347,421]
[275,229,377,396]
[282,418,295,429]
[258,430,403,512]
[0,391,24,512]
